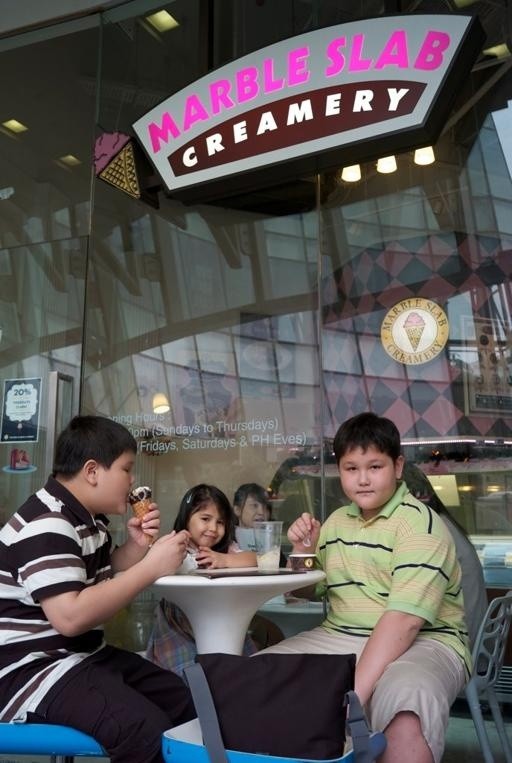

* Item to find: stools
[163,714,388,762]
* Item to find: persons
[0,410,197,763]
[230,480,311,605]
[397,461,499,684]
[144,482,260,685]
[243,409,478,761]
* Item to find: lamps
[444,0,481,13]
[341,145,436,182]
[114,383,170,413]
[482,42,510,59]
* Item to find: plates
[2,465,38,474]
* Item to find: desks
[113,567,327,656]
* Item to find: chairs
[0,720,107,763]
[454,590,509,760]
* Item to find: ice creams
[127,485,153,533]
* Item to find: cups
[252,520,284,570]
[288,554,316,572]
